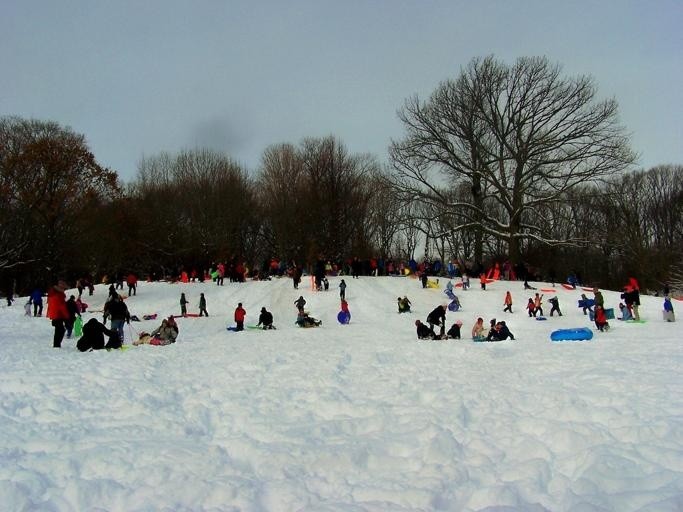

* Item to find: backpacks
[104,328,123,349]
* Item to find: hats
[455,319,462,325]
[58,280,65,288]
